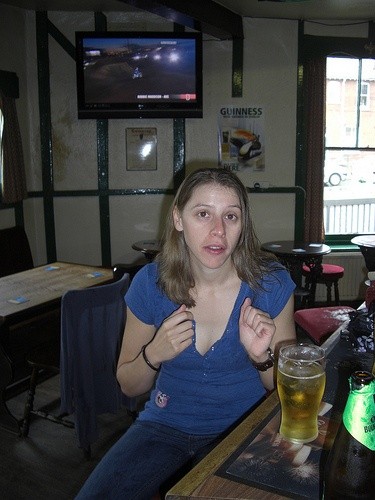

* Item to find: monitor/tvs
[73,30,204,119]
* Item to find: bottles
[321,371,374,500]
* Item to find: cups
[276,343,327,445]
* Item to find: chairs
[22,261,154,461]
[0,225,34,278]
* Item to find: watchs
[252,347,275,371]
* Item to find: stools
[303,263,345,306]
[294,306,357,346]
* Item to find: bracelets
[143,348,159,372]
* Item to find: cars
[324,159,351,186]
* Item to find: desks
[262,240,331,309]
[351,235,375,271]
[133,240,163,263]
[165,301,366,500]
[0,261,114,434]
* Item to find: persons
[74,169,296,500]
